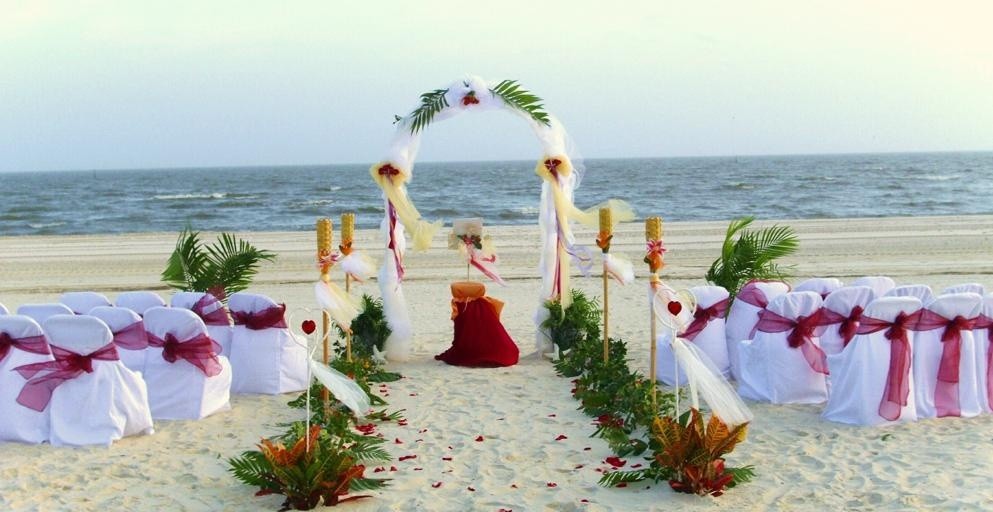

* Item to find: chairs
[938,283,983,296]
[170,291,231,361]
[848,275,896,295]
[1,312,54,447]
[916,292,982,420]
[229,291,310,397]
[17,303,76,330]
[146,306,233,421]
[819,285,881,384]
[114,290,166,317]
[39,313,153,447]
[736,289,829,407]
[655,285,732,389]
[792,277,843,298]
[826,296,923,426]
[974,292,993,415]
[725,279,789,380]
[85,306,147,379]
[58,293,111,317]
[875,284,936,306]
